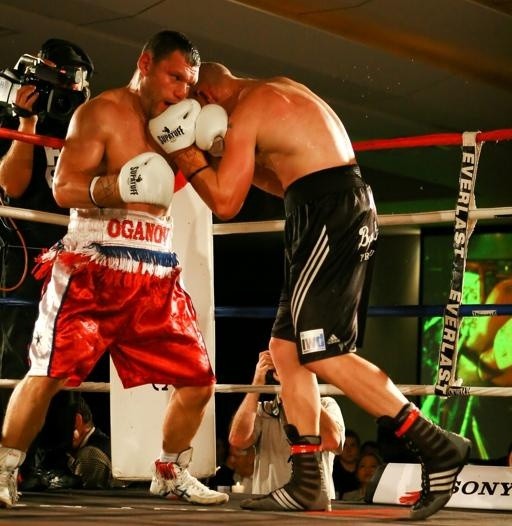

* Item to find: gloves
[119,152,175,208]
[148,98,229,155]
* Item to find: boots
[375,401,472,520]
[239,423,332,511]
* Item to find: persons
[73,395,129,488]
[0,39,94,491]
[228,350,346,500]
[148,61,472,521]
[207,430,387,499]
[1,31,229,508]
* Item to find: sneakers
[36,462,82,491]
[0,447,27,508]
[149,447,229,506]
